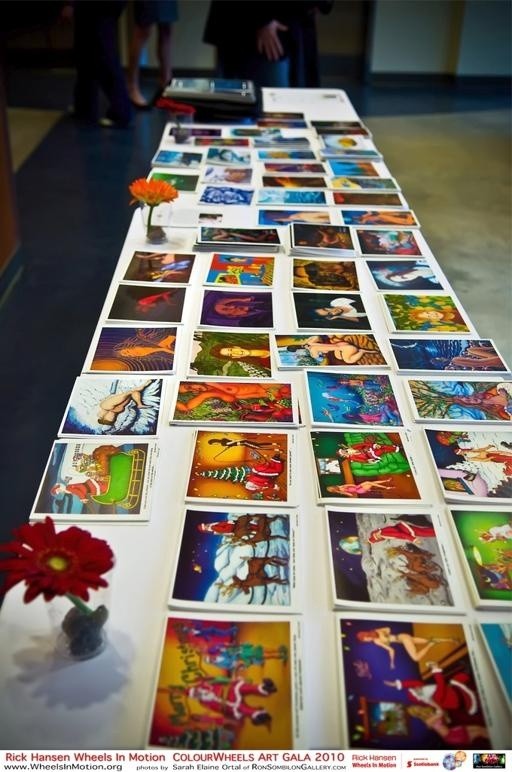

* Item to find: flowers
[128,179,178,232]
[0,517,115,611]
[156,99,194,131]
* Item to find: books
[162,77,255,103]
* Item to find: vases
[139,201,172,245]
[45,589,108,662]
[169,113,193,146]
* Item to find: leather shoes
[127,96,150,114]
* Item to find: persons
[336,443,400,463]
[480,524,512,543]
[185,677,277,724]
[97,379,156,425]
[451,386,512,421]
[408,307,467,325]
[342,404,400,425]
[287,341,380,364]
[212,293,273,327]
[385,660,482,715]
[300,298,368,322]
[202,0,331,88]
[453,444,512,463]
[202,228,243,242]
[175,381,291,412]
[128,0,180,113]
[50,477,115,502]
[263,211,330,225]
[67,0,132,131]
[113,329,175,367]
[326,479,397,497]
[208,439,282,452]
[407,699,490,744]
[338,379,385,393]
[204,645,287,670]
[352,211,415,226]
[195,520,259,538]
[372,259,440,287]
[210,343,271,368]
[356,627,460,669]
